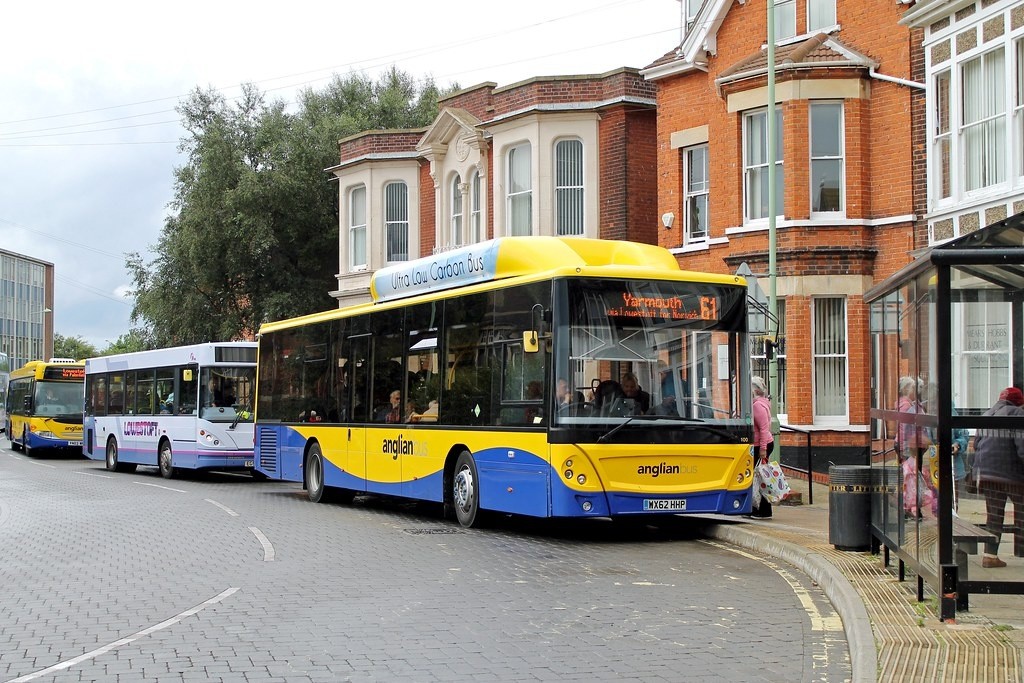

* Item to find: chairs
[138,400,173,415]
[449,397,487,427]
[372,401,391,423]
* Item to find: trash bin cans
[827,461,884,552]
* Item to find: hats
[999,387,1024,407]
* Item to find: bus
[4,357,87,457]
[255,236,783,530]
[0,353,8,430]
[82,341,260,482]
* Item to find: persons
[555,377,574,416]
[646,395,681,417]
[751,376,776,519]
[652,361,690,417]
[375,390,438,423]
[42,388,61,404]
[202,376,227,408]
[968,385,1024,568]
[926,400,970,513]
[893,376,930,522]
[611,374,652,414]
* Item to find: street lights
[28,307,53,363]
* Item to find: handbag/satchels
[751,457,762,509]
[901,455,935,510]
[755,456,791,504]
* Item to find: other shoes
[742,512,773,519]
[982,555,1007,567]
[904,509,916,522]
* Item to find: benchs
[925,511,997,613]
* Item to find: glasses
[392,397,400,400]
